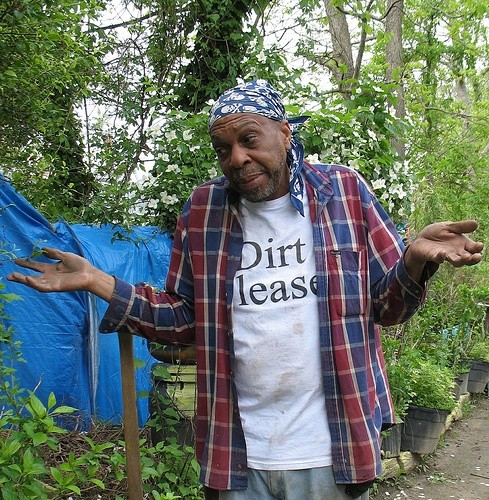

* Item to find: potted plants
[457,368,469,394]
[403,377,450,454]
[457,341,489,393]
[382,371,406,457]
[452,376,463,401]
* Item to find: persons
[6,79,484,500]
